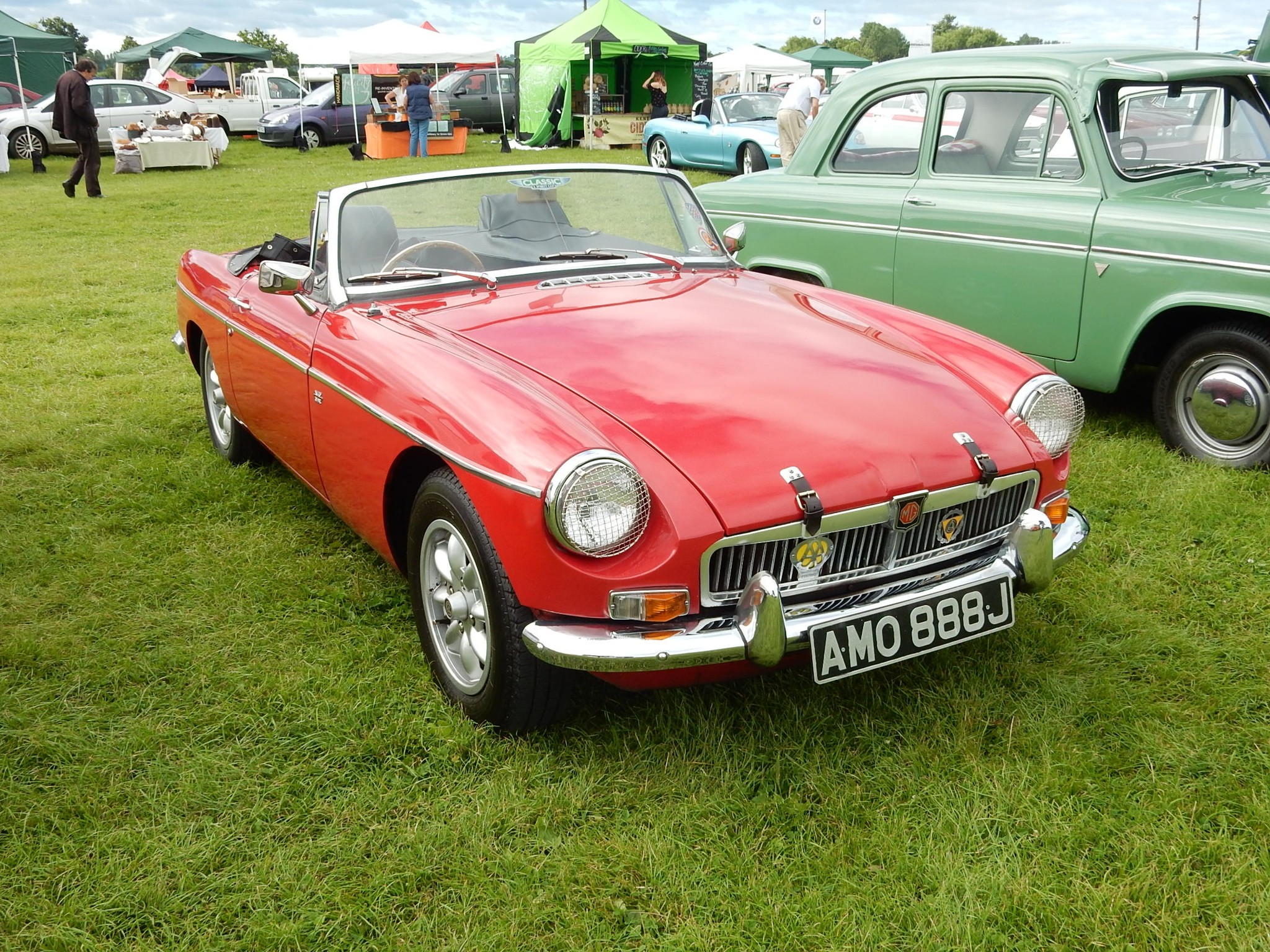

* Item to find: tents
[706,43,812,94]
[297,18,508,151]
[514,0,707,147]
[0,10,78,173]
[115,27,274,96]
[789,45,872,90]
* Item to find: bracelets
[391,105,394,108]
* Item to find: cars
[643,92,812,177]
[0,46,201,160]
[256,75,404,149]
[0,82,42,110]
[173,164,1089,734]
[690,44,1270,471]
[1047,84,1234,158]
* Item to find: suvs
[425,65,515,131]
[850,91,1055,159]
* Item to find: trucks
[189,73,309,137]
[299,67,338,94]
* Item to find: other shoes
[62,179,75,198]
[98,194,106,198]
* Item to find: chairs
[92,92,103,107]
[112,90,119,104]
[731,98,756,118]
[691,99,713,121]
[309,205,419,281]
[462,78,485,94]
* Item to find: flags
[809,11,825,27]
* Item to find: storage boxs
[572,91,608,102]
[156,118,181,126]
[384,108,397,113]
[622,144,641,150]
[190,115,207,127]
[166,78,239,99]
[367,114,386,123]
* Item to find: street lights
[0,37,34,151]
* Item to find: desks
[571,113,691,149]
[107,128,229,160]
[0,134,10,174]
[364,118,472,159]
[136,141,214,171]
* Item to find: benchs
[835,137,992,176]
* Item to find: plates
[181,123,201,135]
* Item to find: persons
[384,75,411,121]
[777,76,825,167]
[52,59,107,199]
[420,66,433,86]
[404,72,435,158]
[642,70,669,120]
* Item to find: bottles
[582,98,623,115]
[643,103,692,114]
